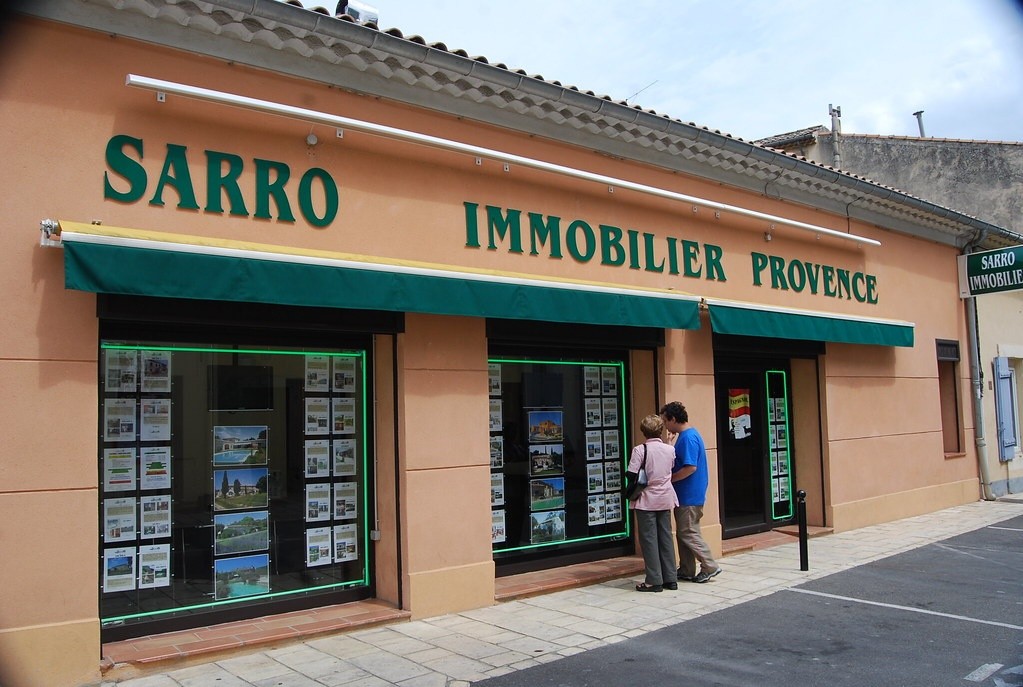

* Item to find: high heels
[663,581,677,590]
[636,583,663,592]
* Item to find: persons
[661,402,722,582]
[627,414,680,592]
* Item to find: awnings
[702,297,915,348]
[57,221,702,329]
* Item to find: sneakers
[677,568,693,581]
[693,567,722,583]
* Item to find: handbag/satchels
[625,444,648,501]
